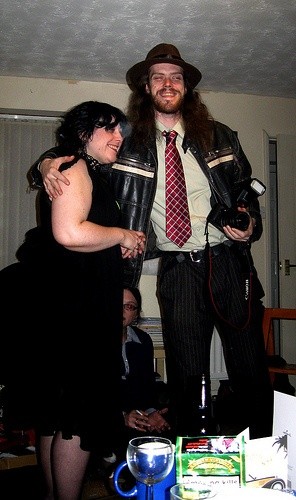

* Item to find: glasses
[123,304,139,311]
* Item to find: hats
[126,43,202,94]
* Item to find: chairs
[262,308,296,385]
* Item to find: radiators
[209,326,229,380]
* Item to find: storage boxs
[175,436,246,500]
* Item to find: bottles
[191,374,222,436]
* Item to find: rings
[138,239,142,244]
[135,426,138,429]
[135,419,139,424]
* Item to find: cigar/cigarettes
[136,410,144,417]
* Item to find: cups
[170,482,217,500]
[114,443,177,500]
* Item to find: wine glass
[125,437,175,500]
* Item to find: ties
[161,130,193,248]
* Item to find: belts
[161,238,236,263]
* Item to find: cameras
[207,202,250,233]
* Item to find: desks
[153,347,165,381]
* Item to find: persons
[30,43,275,440]
[122,284,174,452]
[2,101,146,500]
